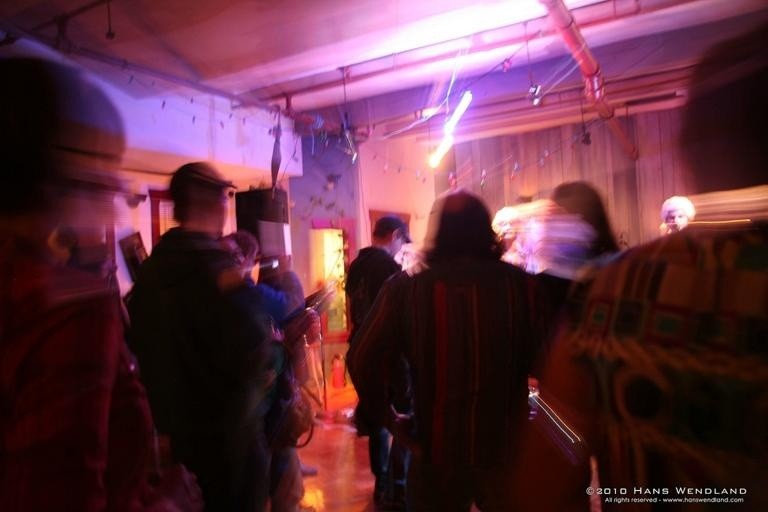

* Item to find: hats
[375,216,412,245]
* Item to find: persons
[0,17,768,510]
